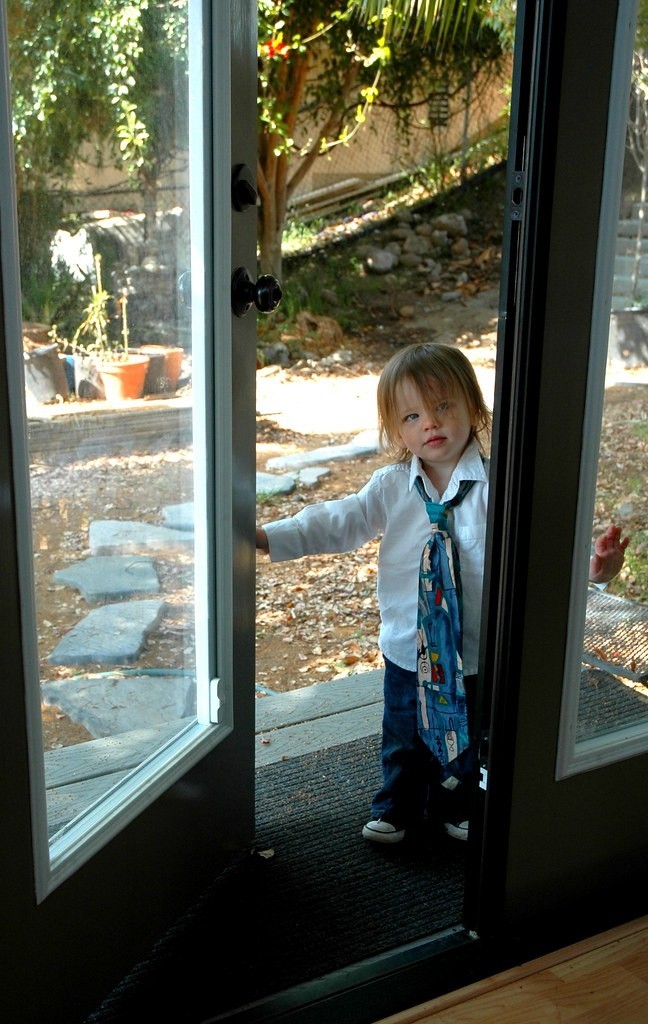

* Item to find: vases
[121,344,185,395]
[21,322,95,404]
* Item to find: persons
[256,343,630,843]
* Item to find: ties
[413,449,485,769]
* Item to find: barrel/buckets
[141,344,184,391]
[23,343,70,404]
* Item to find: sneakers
[437,814,470,840]
[361,815,407,844]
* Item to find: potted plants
[46,250,151,401]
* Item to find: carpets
[48,671,648,1024]
